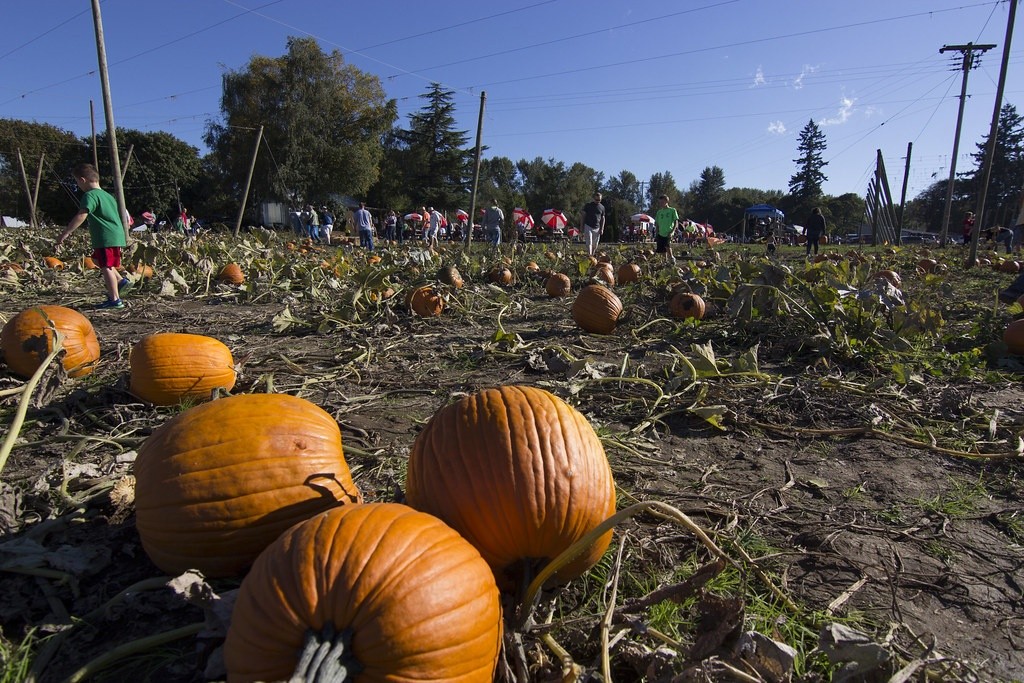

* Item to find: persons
[580,193,606,255]
[421,206,441,247]
[654,195,679,264]
[57,163,131,309]
[177,208,198,236]
[629,222,635,234]
[482,198,504,245]
[353,203,374,250]
[445,220,453,241]
[980,227,1014,254]
[143,207,164,233]
[305,205,322,243]
[461,220,468,243]
[686,221,695,233]
[319,205,336,244]
[767,232,777,252]
[382,210,403,242]
[803,208,826,255]
[998,271,1024,306]
[963,212,976,244]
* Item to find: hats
[321,206,328,210]
[419,207,425,211]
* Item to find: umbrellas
[541,208,568,229]
[405,213,422,221]
[568,226,579,237]
[631,213,655,223]
[513,207,534,230]
[456,209,469,221]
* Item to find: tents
[743,204,784,243]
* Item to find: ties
[427,207,434,213]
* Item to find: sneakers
[118,279,131,297]
[95,298,124,309]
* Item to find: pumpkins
[1,305,238,404]
[133,385,616,683]
[0,240,1024,358]
